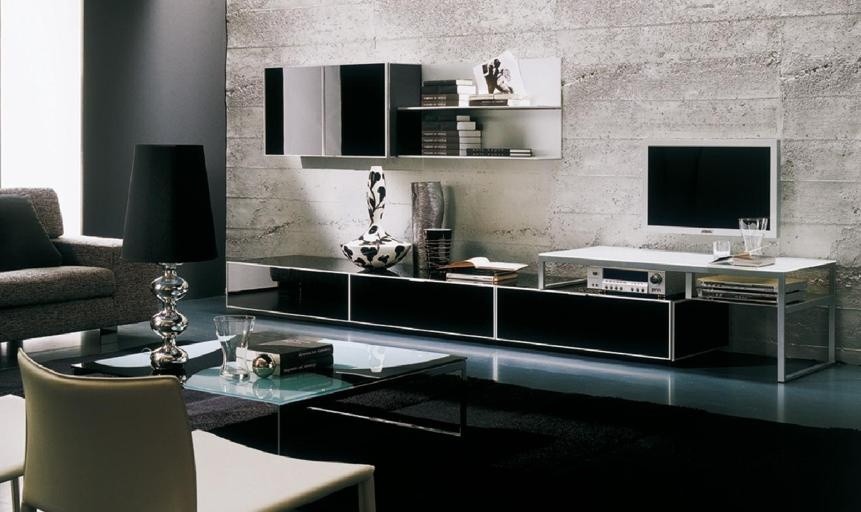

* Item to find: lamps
[122,145,219,386]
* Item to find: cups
[368,346,386,373]
[424,228,453,271]
[214,315,256,378]
[713,239,730,261]
[739,217,770,255]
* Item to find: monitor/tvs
[641,137,780,239]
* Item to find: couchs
[0,187,161,341]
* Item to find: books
[421,78,534,157]
[446,255,529,282]
[236,335,333,376]
[698,274,809,305]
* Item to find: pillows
[1,193,64,270]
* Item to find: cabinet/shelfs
[264,62,563,161]
[227,246,837,386]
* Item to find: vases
[468,92,509,107]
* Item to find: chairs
[0,346,376,512]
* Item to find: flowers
[483,58,515,94]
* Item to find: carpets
[206,368,860,511]
